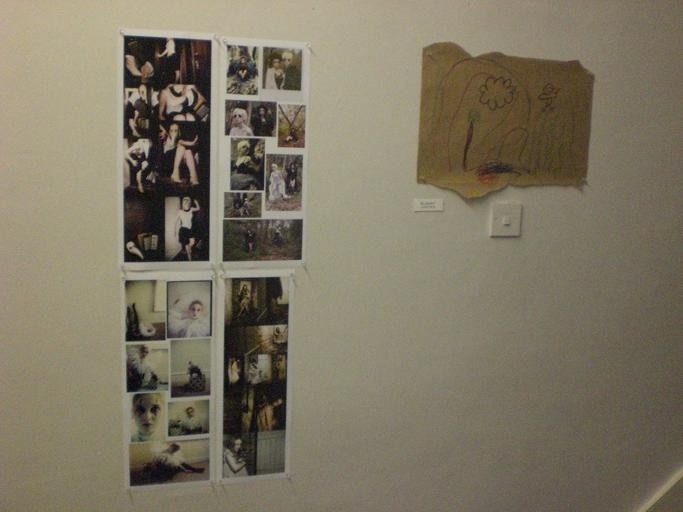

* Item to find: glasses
[233,114,242,118]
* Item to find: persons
[125,285,289,482]
[125,36,301,262]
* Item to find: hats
[257,104,268,114]
